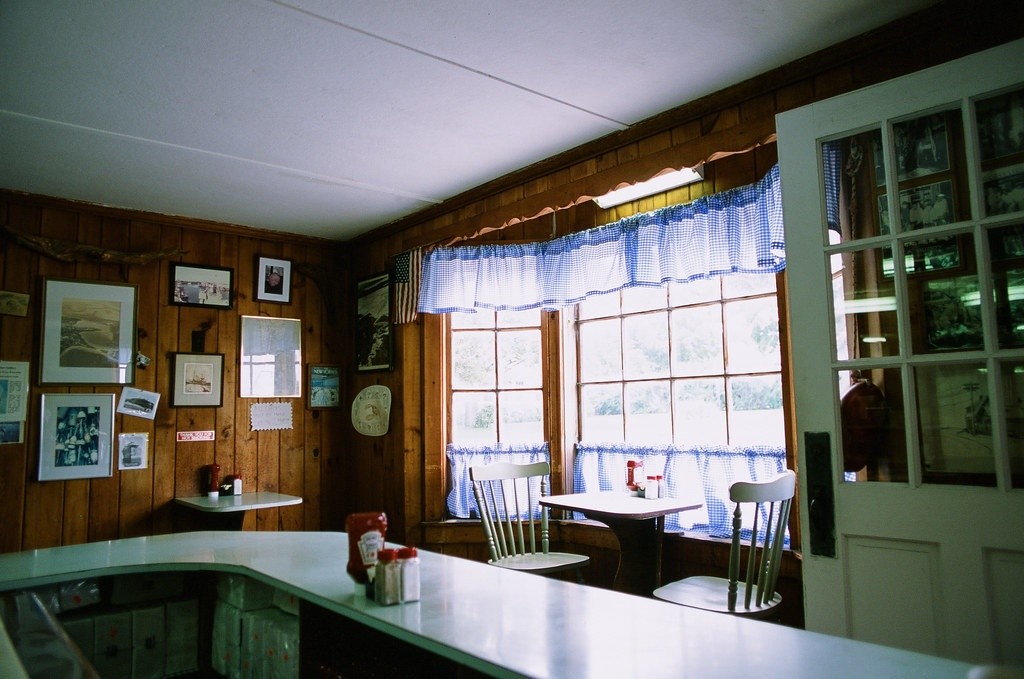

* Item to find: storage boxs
[0,571,200,679]
[215,570,275,612]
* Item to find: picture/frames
[353,269,394,374]
[37,275,140,388]
[307,363,345,411]
[36,392,116,483]
[862,88,1024,488]
[171,352,225,408]
[253,254,293,306]
[239,314,303,399]
[168,261,234,310]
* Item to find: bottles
[396,546,420,604]
[209,463,220,492]
[375,549,398,606]
[231,475,243,496]
[626,460,666,499]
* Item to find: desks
[539,490,703,599]
[175,490,303,532]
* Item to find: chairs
[470,461,591,586]
[653,469,798,624]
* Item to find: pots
[839,369,889,472]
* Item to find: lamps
[592,159,705,210]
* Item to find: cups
[345,512,388,597]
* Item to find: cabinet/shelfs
[774,36,1024,669]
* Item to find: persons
[881,194,950,230]
[987,182,1024,210]
